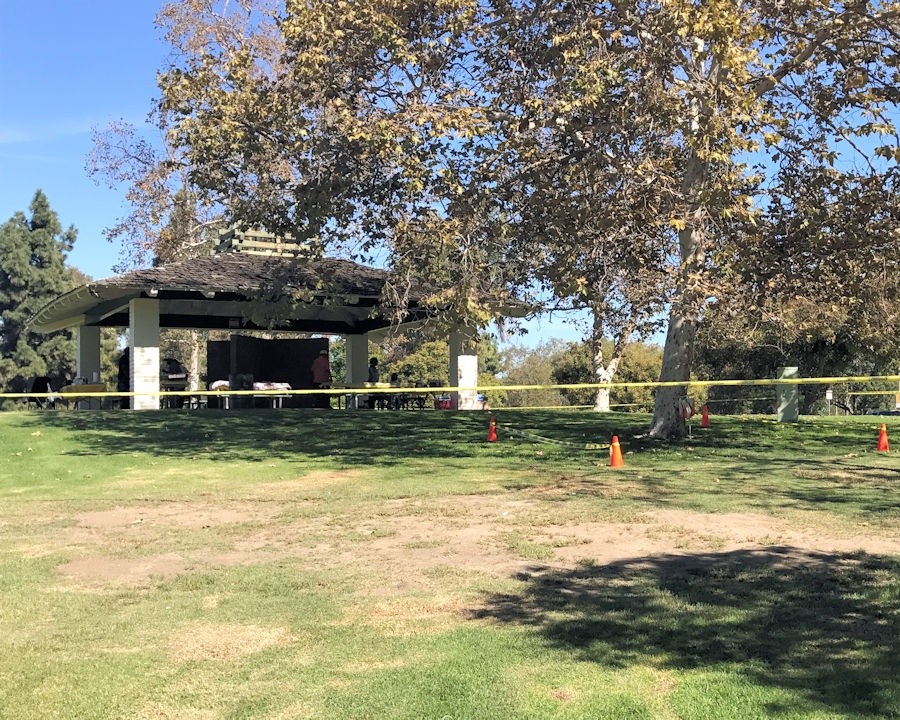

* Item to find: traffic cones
[610,435,625,467]
[701,405,711,427]
[876,423,890,453]
[487,417,498,442]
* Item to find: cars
[478,395,490,401]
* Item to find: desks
[60,384,106,410]
[321,381,416,411]
[160,379,190,409]
[209,380,292,408]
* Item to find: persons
[312,350,333,409]
[367,357,380,409]
[118,346,130,409]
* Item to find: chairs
[20,374,88,410]
[675,394,721,450]
[227,373,254,409]
